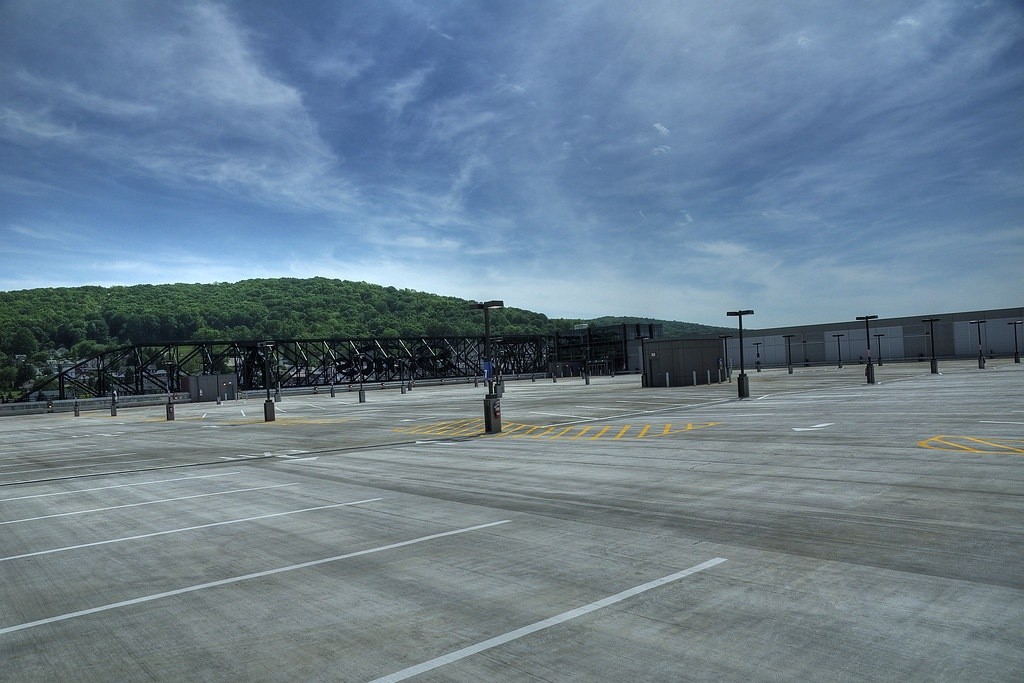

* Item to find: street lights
[72,378,79,417]
[257,341,277,422]
[856,315,879,383]
[752,342,763,373]
[162,361,175,420]
[921,318,941,374]
[354,354,366,403]
[833,334,844,368]
[1007,321,1023,363]
[873,334,884,366]
[970,321,986,369]
[107,369,117,417]
[469,300,505,435]
[782,335,795,374]
[726,310,755,399]
[719,335,732,377]
